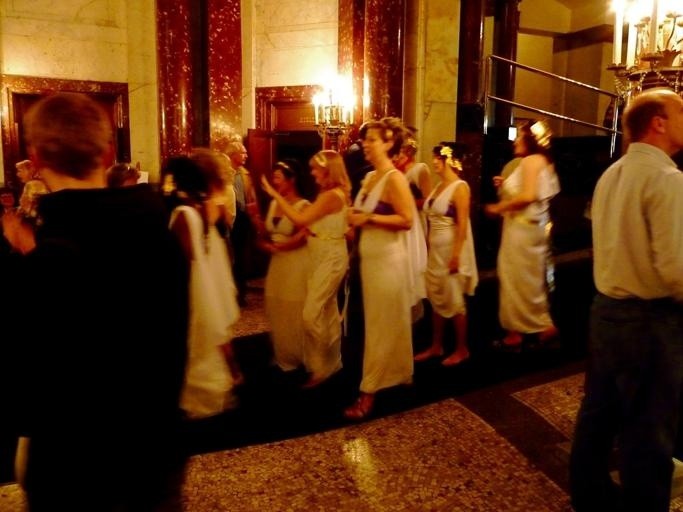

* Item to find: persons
[261,149,353,388]
[394,135,433,322]
[569,87,683,509]
[419,141,479,365]
[485,119,562,345]
[255,161,312,371]
[1,143,260,418]
[341,117,423,419]
[0,94,190,512]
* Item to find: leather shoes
[441,350,470,367]
[493,335,523,347]
[304,363,344,388]
[414,348,445,362]
[539,326,559,343]
[343,394,381,418]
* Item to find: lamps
[312,87,354,151]
[606,1,683,108]
